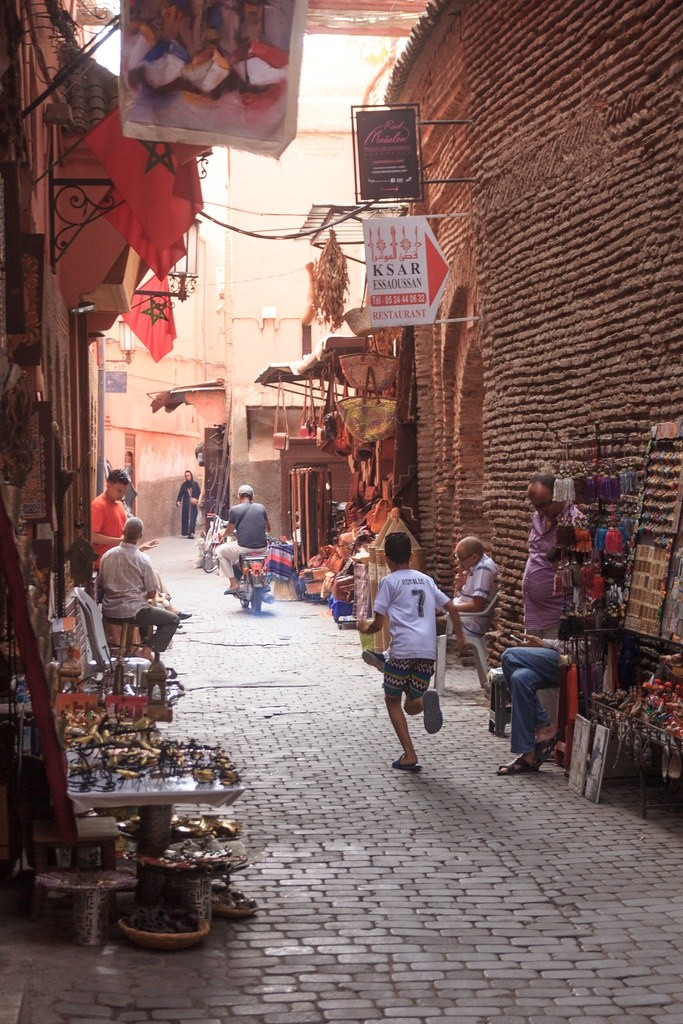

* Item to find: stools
[34,872,138,946]
[553,664,602,778]
[486,667,512,737]
[104,620,153,654]
[135,858,249,925]
[32,817,119,922]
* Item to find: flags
[84,105,204,250]
[66,145,188,281]
[122,274,178,363]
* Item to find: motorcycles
[228,530,274,614]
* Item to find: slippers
[496,757,539,775]
[533,731,561,768]
[263,584,269,591]
[422,690,443,733]
[391,753,421,770]
[223,589,239,595]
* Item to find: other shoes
[362,649,386,673]
[177,624,183,628]
[188,533,194,538]
[177,611,192,620]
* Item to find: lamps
[87,331,105,337]
[68,301,95,320]
[44,103,74,125]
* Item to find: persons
[519,474,585,726]
[495,545,599,776]
[357,531,470,773]
[215,485,271,595]
[175,471,201,540]
[362,536,497,673]
[96,516,179,662]
[119,498,194,628]
[88,469,138,655]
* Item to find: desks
[66,732,245,911]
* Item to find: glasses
[455,552,475,562]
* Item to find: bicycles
[204,513,229,573]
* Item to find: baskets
[209,883,258,916]
[119,912,210,951]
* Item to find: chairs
[434,592,499,696]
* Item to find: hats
[238,485,254,497]
